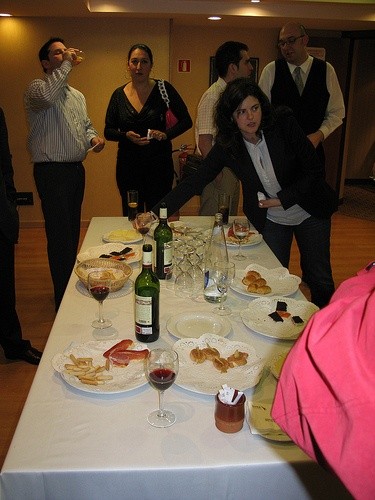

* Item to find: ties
[294,67,304,96]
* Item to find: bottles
[152,202,173,280]
[134,243,160,343]
[204,213,229,303]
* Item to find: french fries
[65,354,113,386]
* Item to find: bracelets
[315,131,324,142]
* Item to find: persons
[103,43,194,218]
[131,78,338,310]
[257,22,346,184]
[270,259,375,500]
[0,108,45,366]
[194,41,254,215]
[23,36,106,315]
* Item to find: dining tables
[1,216,352,500]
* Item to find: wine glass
[212,261,236,316]
[136,212,152,248]
[146,348,179,428]
[233,218,251,261]
[164,235,206,303]
[88,271,113,329]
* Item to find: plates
[165,342,264,395]
[166,311,233,340]
[76,243,142,268]
[211,227,263,246]
[241,297,320,339]
[230,265,300,297]
[102,229,144,244]
[61,341,152,393]
[250,399,294,442]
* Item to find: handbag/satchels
[158,80,177,129]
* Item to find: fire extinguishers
[171,144,197,180]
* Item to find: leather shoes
[6,347,43,365]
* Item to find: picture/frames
[210,56,258,88]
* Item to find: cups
[127,190,138,222]
[73,51,87,64]
[218,193,230,224]
[214,390,246,433]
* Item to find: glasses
[278,34,305,48]
